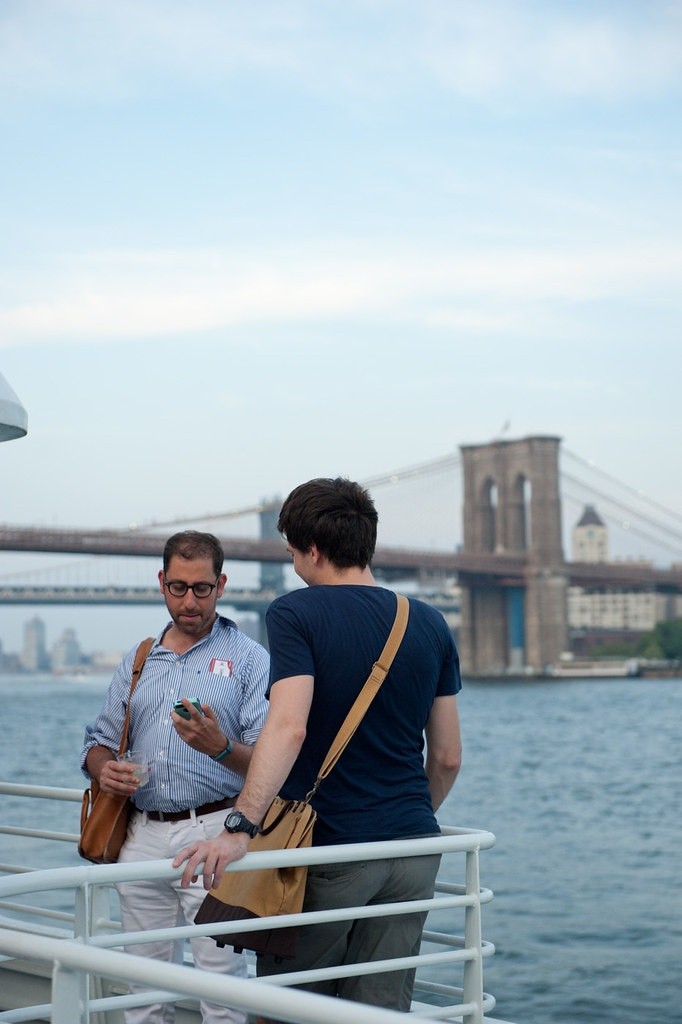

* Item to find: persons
[172,477,462,1024]
[78,529,270,1023]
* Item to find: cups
[116,750,150,788]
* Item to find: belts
[132,795,238,822]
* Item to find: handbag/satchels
[193,797,316,954]
[78,773,136,865]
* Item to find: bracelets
[210,736,233,762]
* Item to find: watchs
[224,811,258,839]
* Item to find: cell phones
[174,698,203,721]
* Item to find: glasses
[163,573,219,598]
